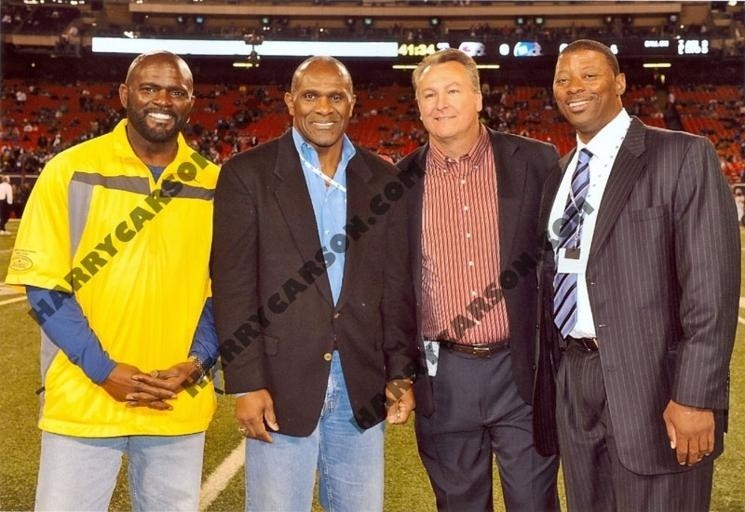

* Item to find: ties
[552,148,593,339]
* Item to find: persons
[210,55,417,512]
[0,0,745,237]
[390,49,561,512]
[529,38,742,512]
[7,50,221,512]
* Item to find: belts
[441,340,511,357]
[575,338,597,352]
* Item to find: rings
[238,426,248,437]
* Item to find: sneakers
[0,230,11,236]
